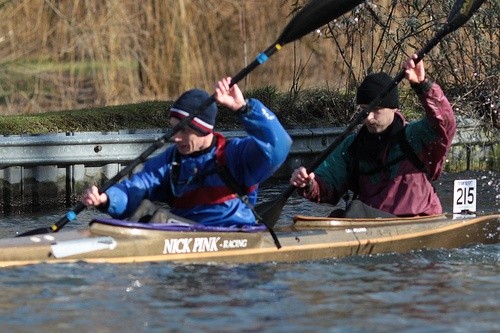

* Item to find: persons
[289,54,456,218]
[81,76,293,228]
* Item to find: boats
[0,211,500,267]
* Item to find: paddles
[14,0,365,237]
[254,0,484,231]
